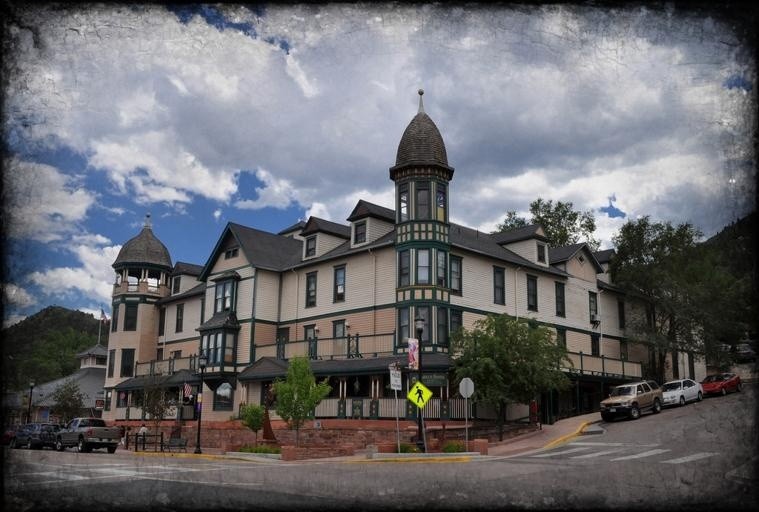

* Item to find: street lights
[192,352,206,454]
[26,376,37,423]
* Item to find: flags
[184,383,193,397]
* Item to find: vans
[10,423,62,450]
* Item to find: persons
[0,417,23,449]
[112,423,133,449]
[138,423,149,448]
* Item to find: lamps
[592,313,601,329]
[345,318,351,331]
[354,376,361,394]
[313,324,320,335]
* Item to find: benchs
[161,437,189,453]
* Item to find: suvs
[600,381,663,422]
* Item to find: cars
[701,373,744,396]
[662,379,705,407]
[3,428,16,448]
[55,417,124,453]
